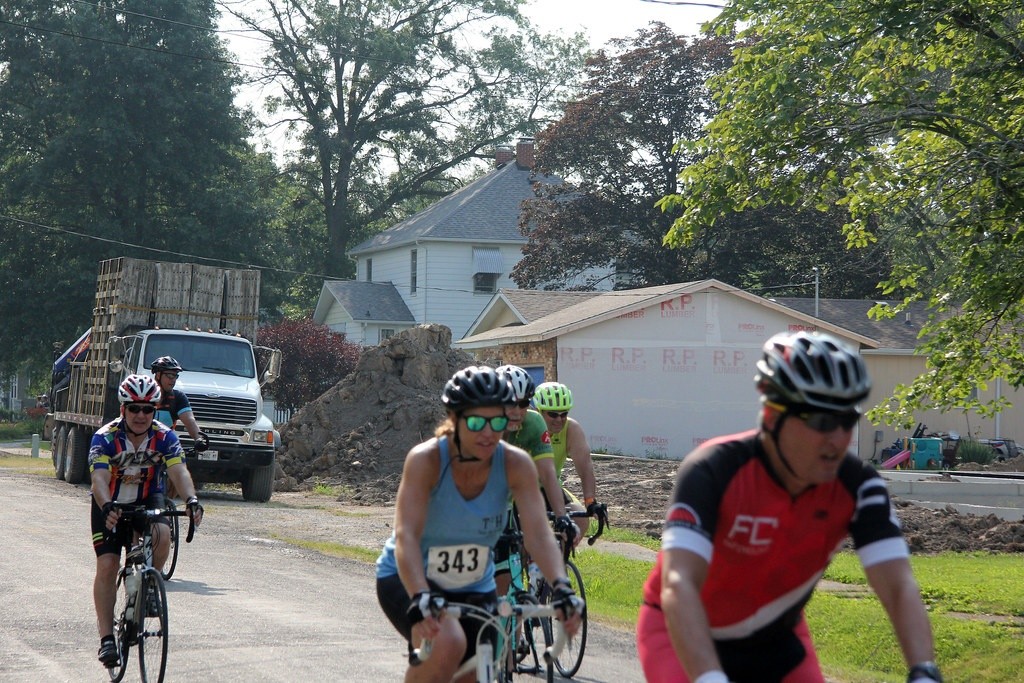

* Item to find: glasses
[459,416,509,432]
[766,400,861,433]
[504,400,530,409]
[159,372,179,380]
[124,404,157,414]
[542,409,569,418]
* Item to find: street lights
[812,266,819,319]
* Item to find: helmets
[755,331,871,413]
[151,356,182,374]
[440,365,516,410]
[532,381,574,411]
[118,374,161,404]
[494,364,535,401]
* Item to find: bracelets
[584,498,593,503]
[186,496,196,503]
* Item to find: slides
[879,449,911,469]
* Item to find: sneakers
[148,592,162,616]
[98,642,116,662]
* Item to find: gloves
[194,440,208,451]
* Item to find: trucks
[48,324,282,505]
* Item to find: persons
[533,381,607,550]
[637,331,944,683]
[492,366,578,669]
[375,366,585,683]
[88,372,204,662]
[36,395,48,408]
[151,356,207,499]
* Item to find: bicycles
[407,586,577,683]
[496,500,612,683]
[104,499,196,682]
[162,431,212,581]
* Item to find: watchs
[907,663,943,683]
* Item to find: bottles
[125,565,143,620]
[527,559,539,596]
